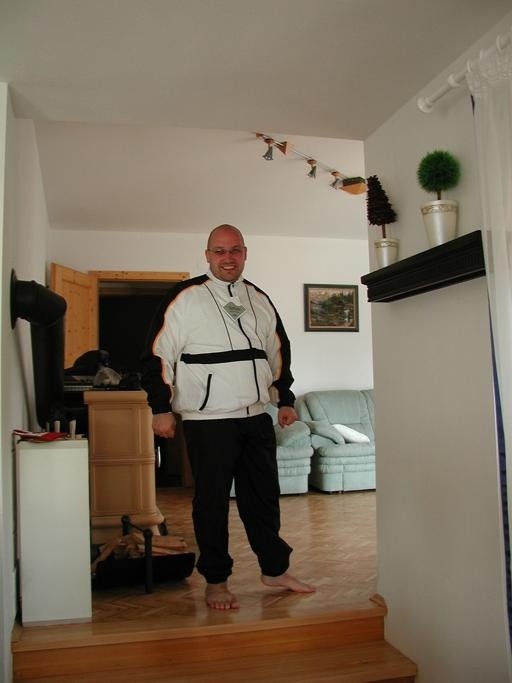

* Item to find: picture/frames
[302,282,361,333]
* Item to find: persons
[142,225,316,610]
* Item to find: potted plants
[366,173,402,270]
[413,146,461,248]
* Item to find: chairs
[227,402,313,498]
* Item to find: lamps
[259,136,346,191]
[340,175,367,195]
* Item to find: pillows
[303,417,346,444]
[332,421,371,442]
[273,417,311,447]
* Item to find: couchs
[292,387,376,496]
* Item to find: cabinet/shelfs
[14,433,94,629]
[82,387,168,537]
